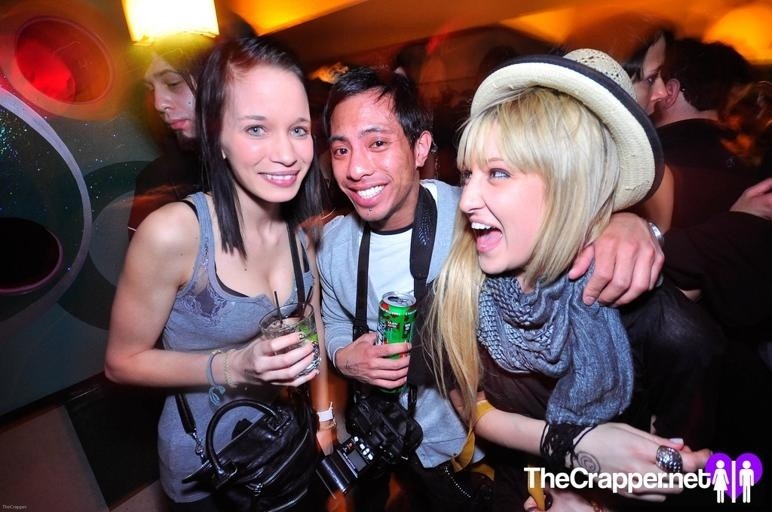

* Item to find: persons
[104,12,772,511]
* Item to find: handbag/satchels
[181,389,324,512]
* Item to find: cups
[259,302,320,379]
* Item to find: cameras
[316,387,423,500]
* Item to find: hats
[470,48,665,213]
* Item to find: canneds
[375,291,417,392]
